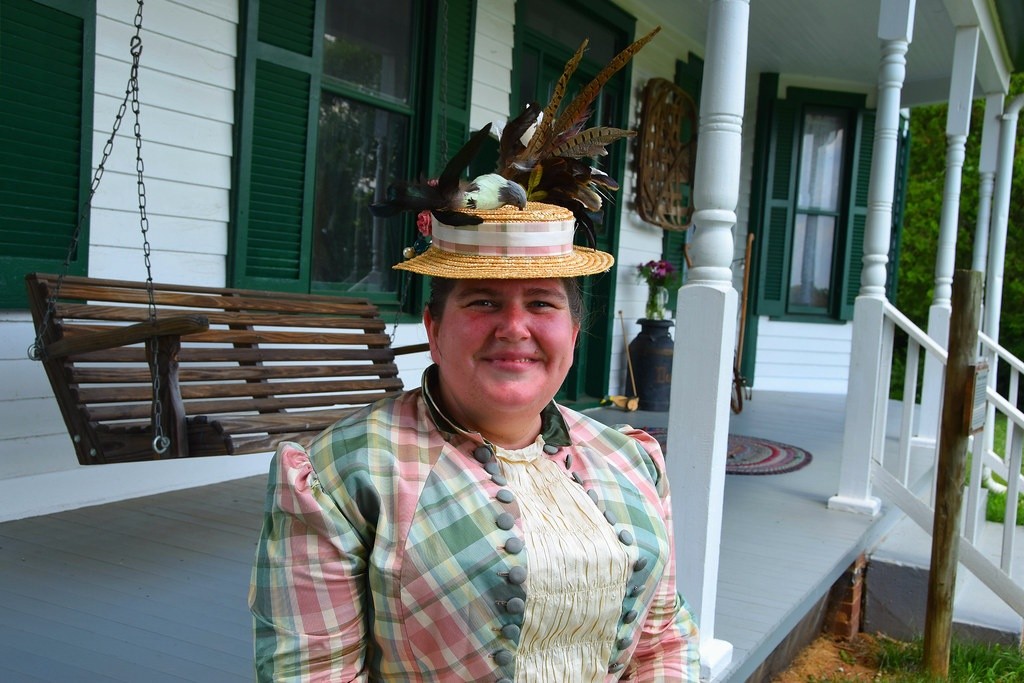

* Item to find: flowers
[638,258,672,320]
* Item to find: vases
[646,286,670,319]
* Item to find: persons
[245,198,701,683]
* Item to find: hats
[393,196,615,276]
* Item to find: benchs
[25,269,407,467]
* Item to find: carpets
[627,426,814,475]
